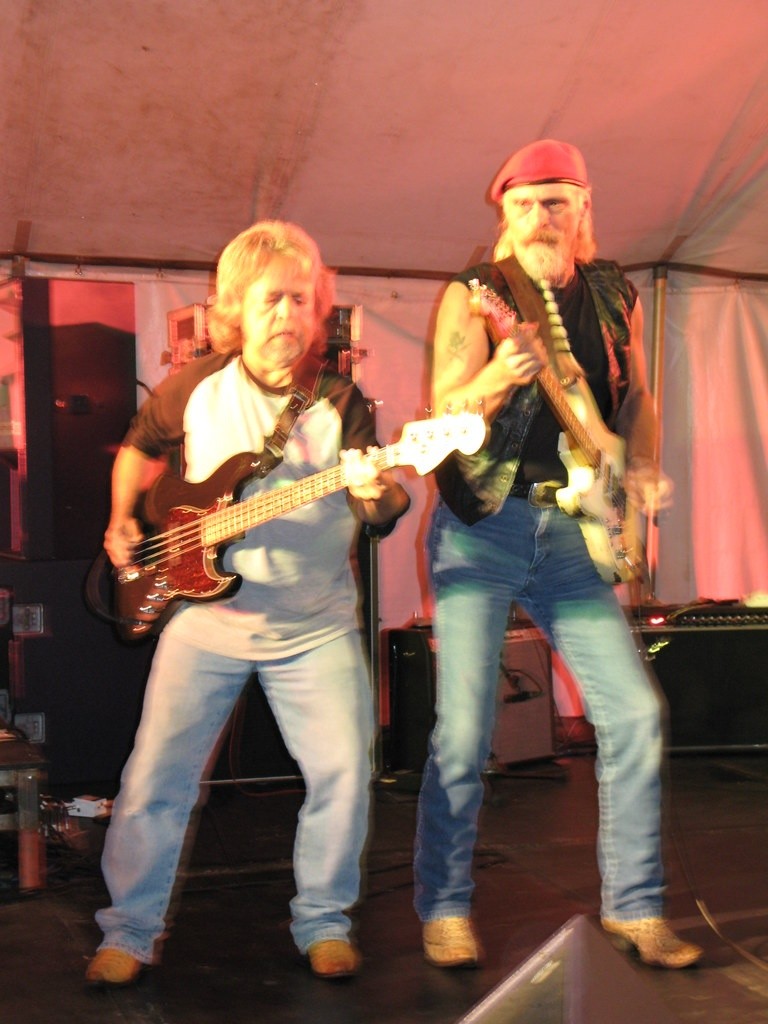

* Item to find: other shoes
[418,913,480,967]
[309,936,358,979]
[85,948,140,987]
[601,913,704,969]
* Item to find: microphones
[504,691,541,703]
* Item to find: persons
[415,139,703,966]
[84,222,410,986]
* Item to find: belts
[507,479,566,509]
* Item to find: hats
[487,139,591,204]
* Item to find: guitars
[468,275,650,583]
[114,395,496,642]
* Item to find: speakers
[388,617,558,776]
[456,917,680,1024]
[624,602,768,754]
